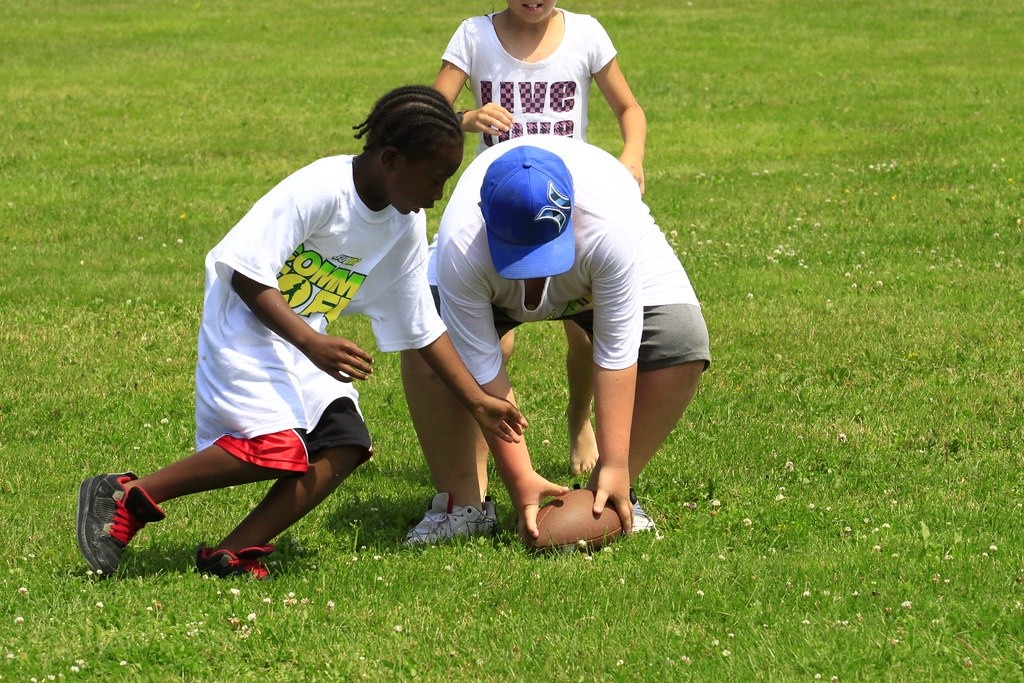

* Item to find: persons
[73,84,528,579]
[431,1,648,481]
[401,133,713,543]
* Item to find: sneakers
[195,541,275,581]
[627,486,655,534]
[401,492,502,548]
[76,470,168,579]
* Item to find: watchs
[455,109,471,122]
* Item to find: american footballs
[525,488,623,557]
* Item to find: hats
[479,144,576,280]
